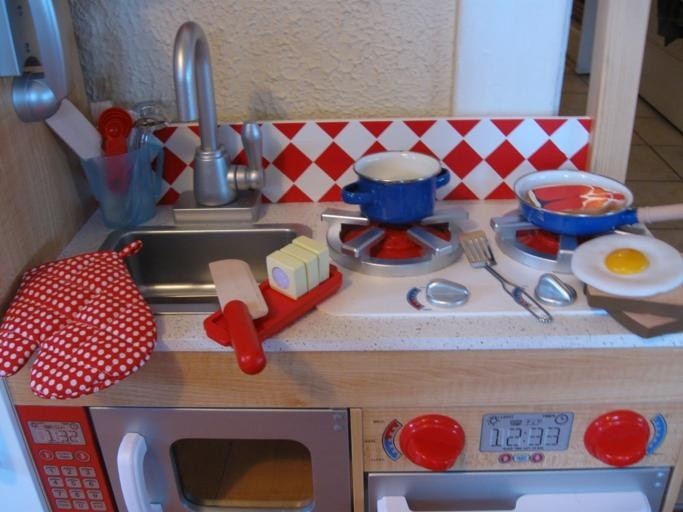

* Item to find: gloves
[0,239,158,401]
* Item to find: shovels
[457,229,552,325]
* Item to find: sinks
[97,222,312,314]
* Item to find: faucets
[170,19,267,224]
